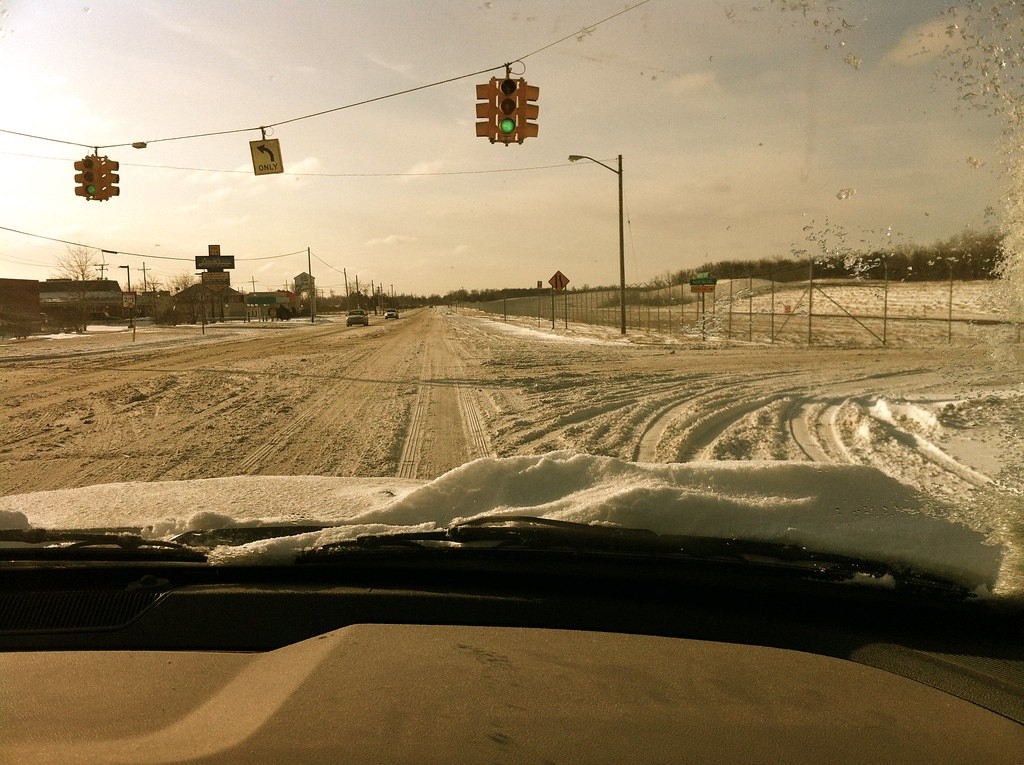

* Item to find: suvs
[345,309,369,327]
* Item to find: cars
[384,308,400,319]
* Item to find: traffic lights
[497,78,518,141]
[73,159,86,197]
[517,80,539,140]
[475,78,496,140]
[82,156,103,200]
[102,158,120,198]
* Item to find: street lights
[567,152,627,334]
[118,264,133,324]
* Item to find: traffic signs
[248,138,284,175]
